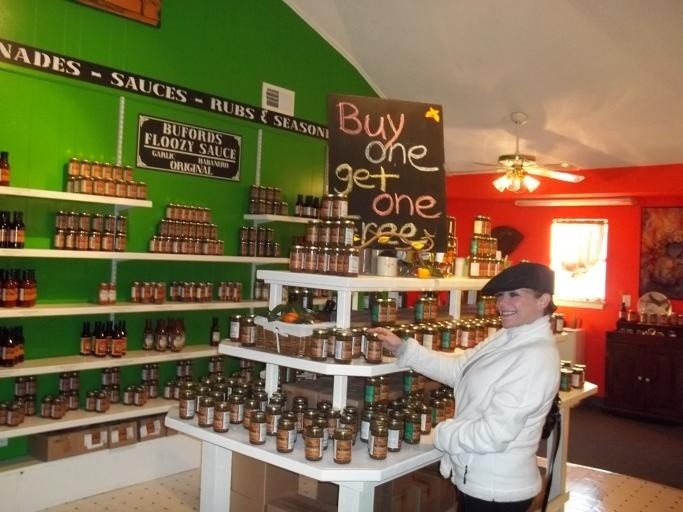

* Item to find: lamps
[492,165,540,193]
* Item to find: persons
[363,262,561,512]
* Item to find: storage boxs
[28,414,173,462]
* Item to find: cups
[373,254,404,277]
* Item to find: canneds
[131,281,167,304]
[248,184,289,216]
[163,361,191,400]
[218,281,242,302]
[123,364,158,406]
[2,377,35,426]
[229,288,503,364]
[238,358,254,372]
[238,225,281,258]
[626,310,683,325]
[468,214,505,279]
[206,357,223,372]
[65,159,147,199]
[255,282,270,300]
[98,282,117,305]
[148,204,224,256]
[40,372,81,419]
[549,313,564,336]
[53,211,127,252]
[169,280,214,302]
[86,367,120,413]
[557,360,586,392]
[178,372,455,465]
[289,191,360,278]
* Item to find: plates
[637,292,672,318]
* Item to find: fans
[472,112,585,183]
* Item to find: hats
[479,262,555,294]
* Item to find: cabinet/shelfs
[165,269,599,512]
[602,319,683,425]
[557,327,585,364]
[0,185,321,512]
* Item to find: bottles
[78,318,127,360]
[361,247,383,276]
[0,211,26,248]
[0,152,10,186]
[0,326,27,367]
[208,316,220,346]
[317,299,336,323]
[294,195,322,217]
[616,302,682,339]
[0,267,36,308]
[141,316,186,354]
